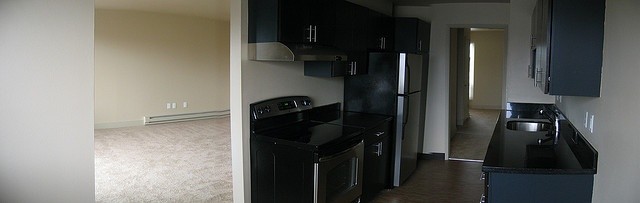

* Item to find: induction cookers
[250,96,365,152]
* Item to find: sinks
[507,121,553,131]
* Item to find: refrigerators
[343,53,423,190]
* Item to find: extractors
[249,42,349,64]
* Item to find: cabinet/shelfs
[534,0,606,97]
[527,15,533,80]
[364,2,398,74]
[531,6,537,51]
[363,114,394,202]
[308,1,361,78]
[398,17,431,55]
[250,1,331,60]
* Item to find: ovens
[250,140,365,203]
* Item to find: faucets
[539,109,554,124]
